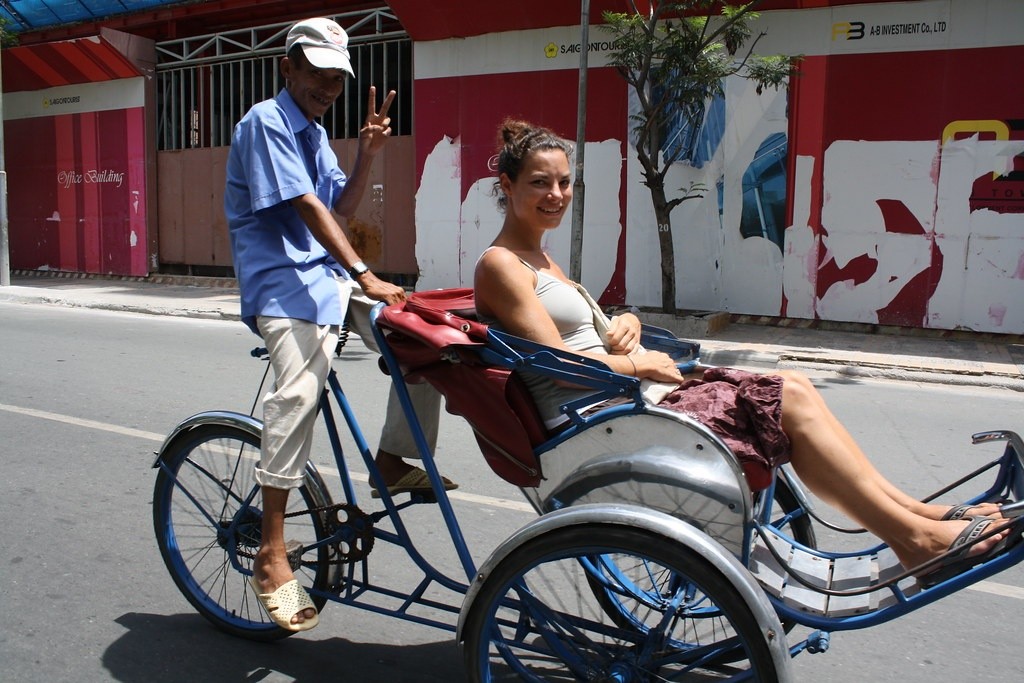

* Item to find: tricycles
[149,280,1024,683]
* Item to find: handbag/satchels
[577,281,680,407]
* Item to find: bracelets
[627,354,641,378]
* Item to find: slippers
[915,522,1016,589]
[942,501,1009,523]
[248,566,319,631]
[369,461,458,498]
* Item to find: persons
[473,122,1023,589]
[222,16,459,630]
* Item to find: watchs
[349,261,370,281]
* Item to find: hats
[284,17,355,78]
